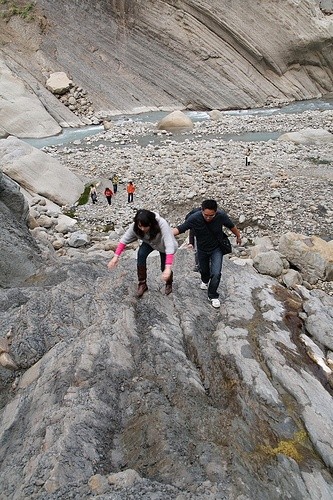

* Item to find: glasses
[202,211,215,218]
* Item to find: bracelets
[238,237,241,240]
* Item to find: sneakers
[193,263,201,272]
[208,296,221,308]
[136,282,148,297]
[200,282,209,290]
[165,283,172,295]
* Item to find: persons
[107,209,178,298]
[188,208,202,273]
[104,188,113,206]
[127,182,135,204]
[112,173,119,196]
[172,200,242,307]
[245,149,251,166]
[90,184,99,205]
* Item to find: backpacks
[185,207,201,220]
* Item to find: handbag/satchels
[198,211,232,255]
